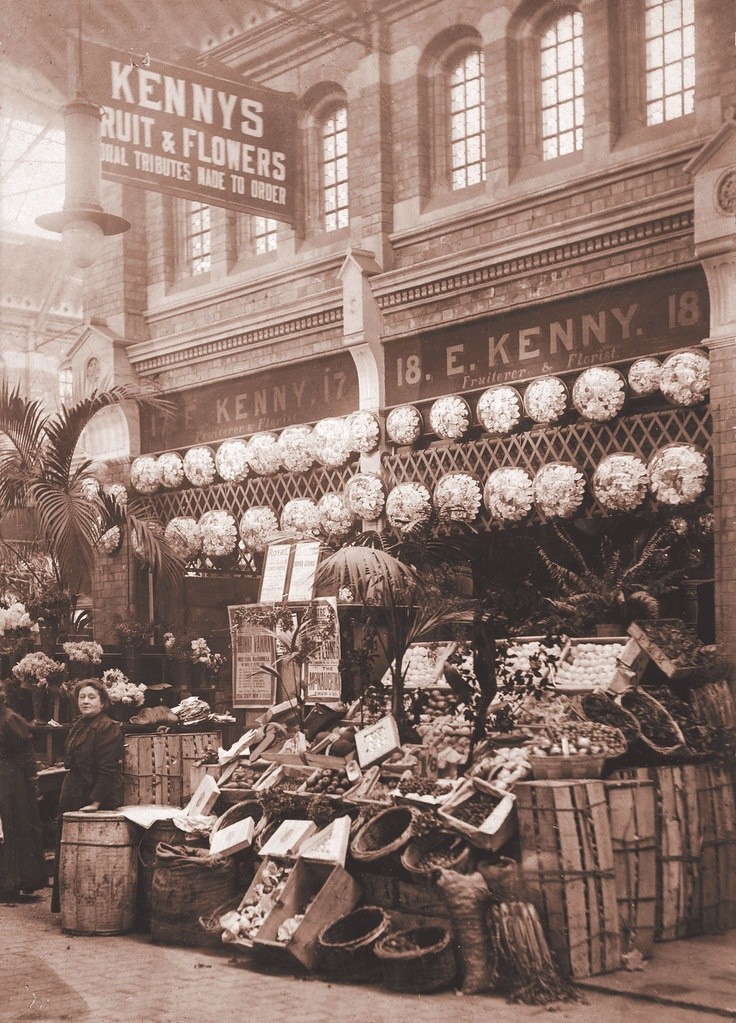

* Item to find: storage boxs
[191,619,716,976]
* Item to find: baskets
[569,692,641,749]
[615,684,685,764]
[148,800,469,994]
[528,752,607,780]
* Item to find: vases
[0,652,10,679]
[111,705,132,721]
[31,690,49,725]
[191,662,207,688]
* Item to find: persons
[0,686,46,902]
[51,681,123,913]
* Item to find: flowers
[64,641,103,665]
[103,669,149,708]
[0,603,32,652]
[12,652,67,688]
[190,640,227,673]
